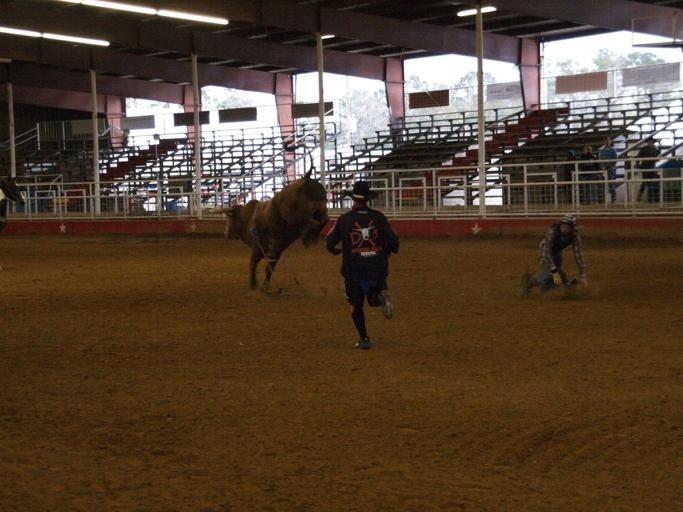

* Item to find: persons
[521,215,587,294]
[564,137,617,205]
[326,181,400,350]
[636,138,660,203]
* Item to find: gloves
[551,268,562,286]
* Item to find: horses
[0,173,26,231]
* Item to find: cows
[208,140,328,290]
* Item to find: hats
[344,180,377,203]
[560,215,577,226]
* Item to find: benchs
[4,107,345,211]
[257,89,680,208]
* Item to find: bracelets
[551,268,558,274]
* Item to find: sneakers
[380,293,394,320]
[354,336,371,350]
